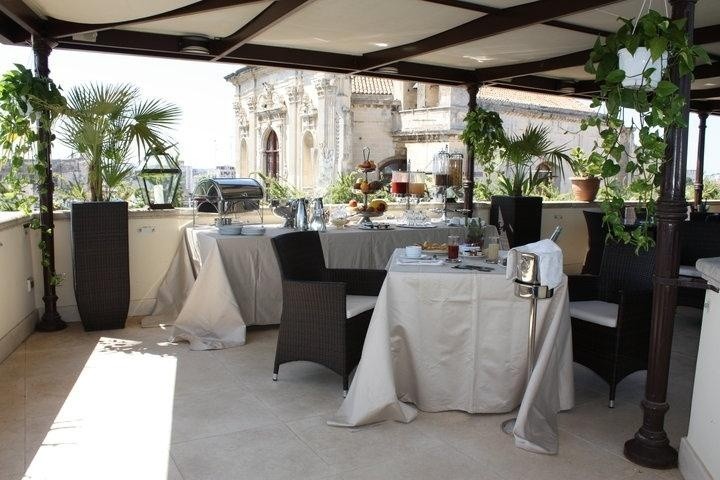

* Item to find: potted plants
[25,81,183,331]
[563,9,713,256]
[490,122,581,250]
[0,64,66,286]
[457,106,504,199]
[568,146,604,200]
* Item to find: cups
[488,236,500,261]
[447,235,460,261]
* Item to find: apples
[349,199,357,207]
[377,203,385,211]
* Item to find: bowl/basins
[405,246,422,258]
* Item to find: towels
[506,239,563,290]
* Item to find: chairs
[271,231,387,398]
[569,206,720,408]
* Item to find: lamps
[137,154,182,209]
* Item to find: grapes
[353,204,367,212]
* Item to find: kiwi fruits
[366,207,374,212]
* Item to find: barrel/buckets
[515,251,544,286]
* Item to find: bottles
[293,197,327,232]
[465,249,482,255]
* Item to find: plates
[218,225,266,236]
[460,254,486,260]
[420,249,449,255]
